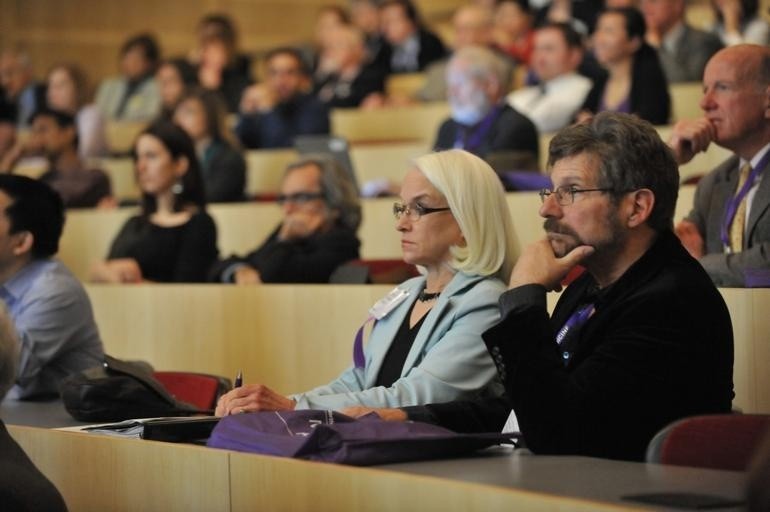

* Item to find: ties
[727,160,754,255]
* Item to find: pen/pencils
[235,370,243,388]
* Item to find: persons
[0,2,769,457]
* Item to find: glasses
[539,183,630,207]
[393,200,453,223]
[273,190,327,208]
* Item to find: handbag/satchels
[62,353,199,423]
[206,408,515,467]
